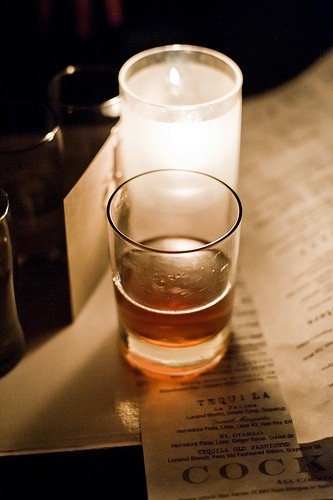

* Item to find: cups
[118,44,243,244]
[106,168,243,377]
